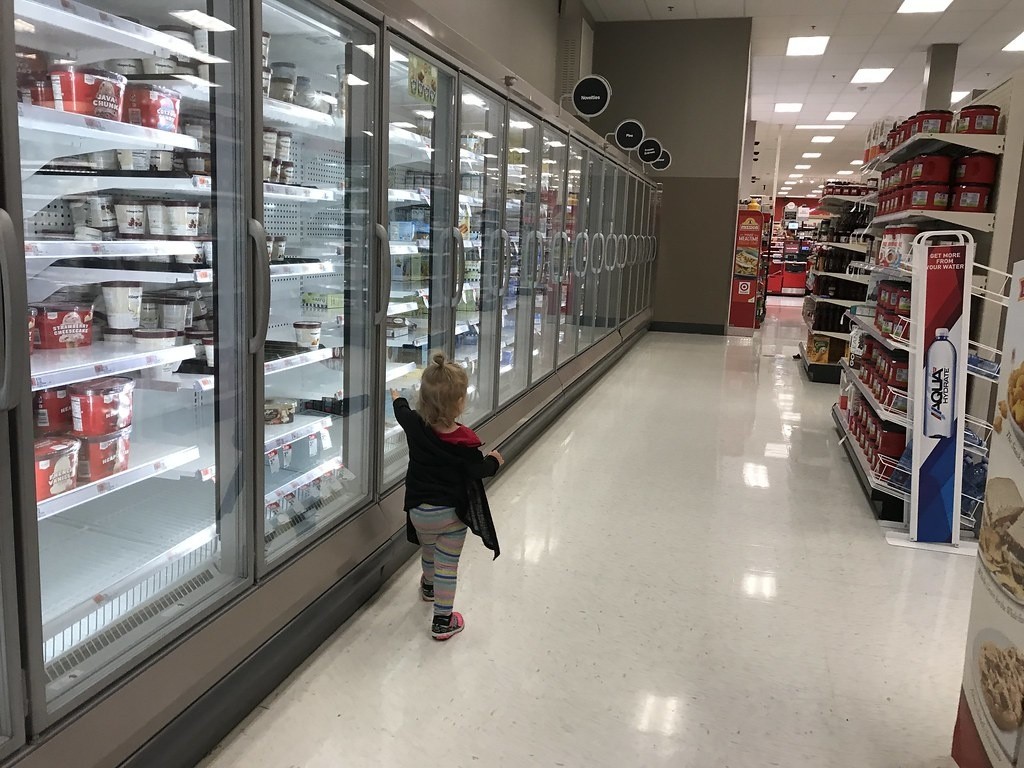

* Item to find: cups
[262,67,273,98]
[275,131,293,162]
[143,285,214,330]
[133,329,179,380]
[164,201,199,236]
[202,337,214,367]
[281,161,294,185]
[271,157,281,183]
[199,203,213,237]
[147,201,170,235]
[263,127,278,160]
[265,235,274,262]
[101,281,143,329]
[203,241,212,268]
[113,199,147,239]
[274,236,287,261]
[262,31,271,67]
[293,321,321,351]
[263,155,273,183]
[85,195,117,233]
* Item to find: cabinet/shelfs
[831,67,1024,530]
[799,171,884,384]
[0,0,665,768]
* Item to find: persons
[817,219,831,242]
[391,352,505,640]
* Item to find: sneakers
[420,574,434,601]
[431,612,465,640]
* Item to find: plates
[972,628,1024,763]
[1007,389,1024,449]
[977,544,1024,607]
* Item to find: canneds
[818,181,869,242]
[847,103,1001,480]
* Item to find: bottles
[820,276,868,301]
[816,248,865,274]
[336,64,345,92]
[865,236,883,265]
[964,428,988,450]
[829,230,869,243]
[815,302,852,334]
[967,353,998,378]
[924,328,956,440]
[335,92,345,120]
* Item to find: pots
[734,249,758,278]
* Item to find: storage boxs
[806,330,830,362]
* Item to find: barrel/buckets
[961,453,989,519]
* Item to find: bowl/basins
[185,331,214,345]
[142,55,178,74]
[151,150,174,171]
[50,64,127,123]
[30,80,55,108]
[122,82,181,134]
[267,63,297,90]
[146,255,173,263]
[185,152,211,175]
[86,147,117,170]
[157,25,195,45]
[105,60,143,75]
[177,330,185,346]
[178,61,198,76]
[123,256,146,263]
[117,149,151,170]
[173,241,205,264]
[269,77,294,103]
[174,152,185,173]
